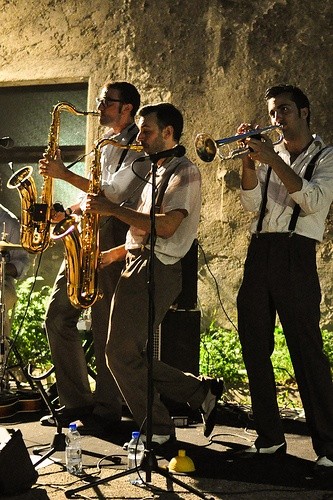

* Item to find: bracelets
[67,208,72,214]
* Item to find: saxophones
[48,137,144,311]
[5,99,101,256]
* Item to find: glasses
[96,97,125,106]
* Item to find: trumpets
[193,121,288,165]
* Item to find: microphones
[135,145,186,162]
[0,136,14,150]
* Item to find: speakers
[152,308,201,426]
[0,426,39,500]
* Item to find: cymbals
[0,232,23,252]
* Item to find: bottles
[128,431,145,470]
[65,424,82,476]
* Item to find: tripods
[4,336,121,470]
[65,163,217,500]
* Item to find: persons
[85,103,225,451]
[0,202,29,390]
[38,81,153,436]
[227,83,333,481]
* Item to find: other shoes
[69,414,121,439]
[39,406,92,428]
[199,377,224,438]
[303,454,333,484]
[234,436,287,469]
[123,431,176,454]
